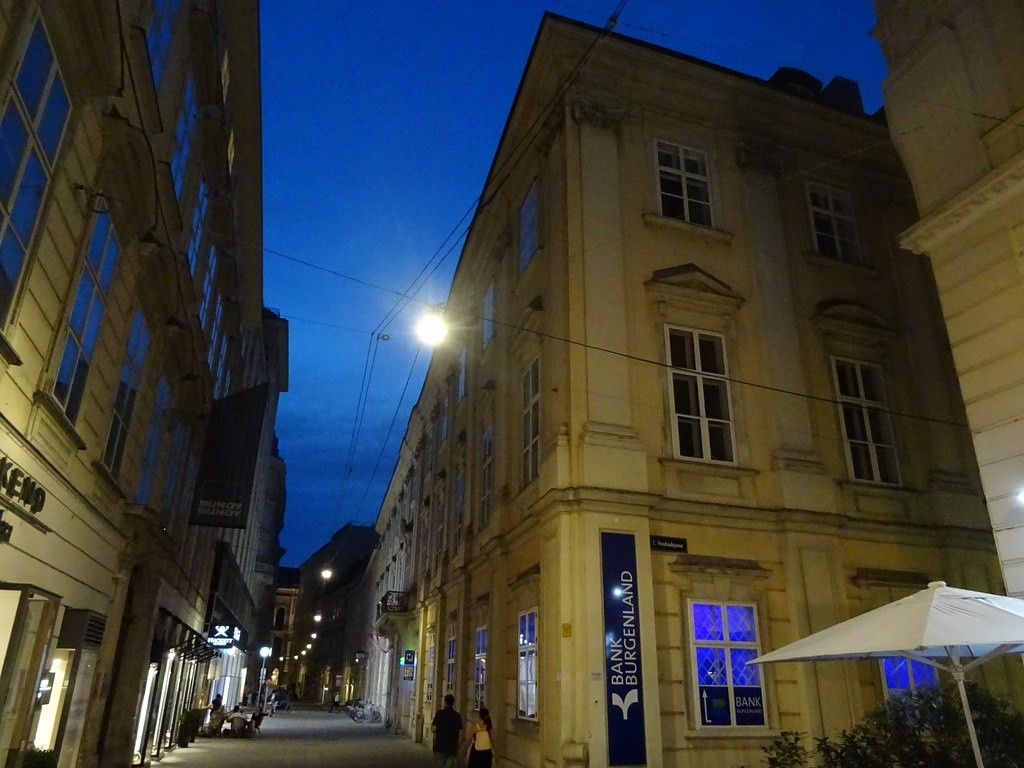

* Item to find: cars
[270,692,291,710]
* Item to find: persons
[250,706,262,736]
[431,694,463,768]
[464,707,495,768]
[328,688,341,713]
[227,705,247,720]
[210,693,223,718]
[267,689,279,717]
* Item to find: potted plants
[177,709,207,748]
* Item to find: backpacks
[475,722,493,750]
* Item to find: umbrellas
[744,580,1024,768]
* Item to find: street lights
[256,647,270,707]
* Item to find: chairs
[231,717,246,738]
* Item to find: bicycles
[353,704,381,724]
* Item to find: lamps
[423,495,431,506]
[458,428,468,447]
[100,103,210,422]
[438,468,447,479]
[528,296,545,312]
[372,519,414,586]
[172,635,222,664]
[480,381,497,392]
